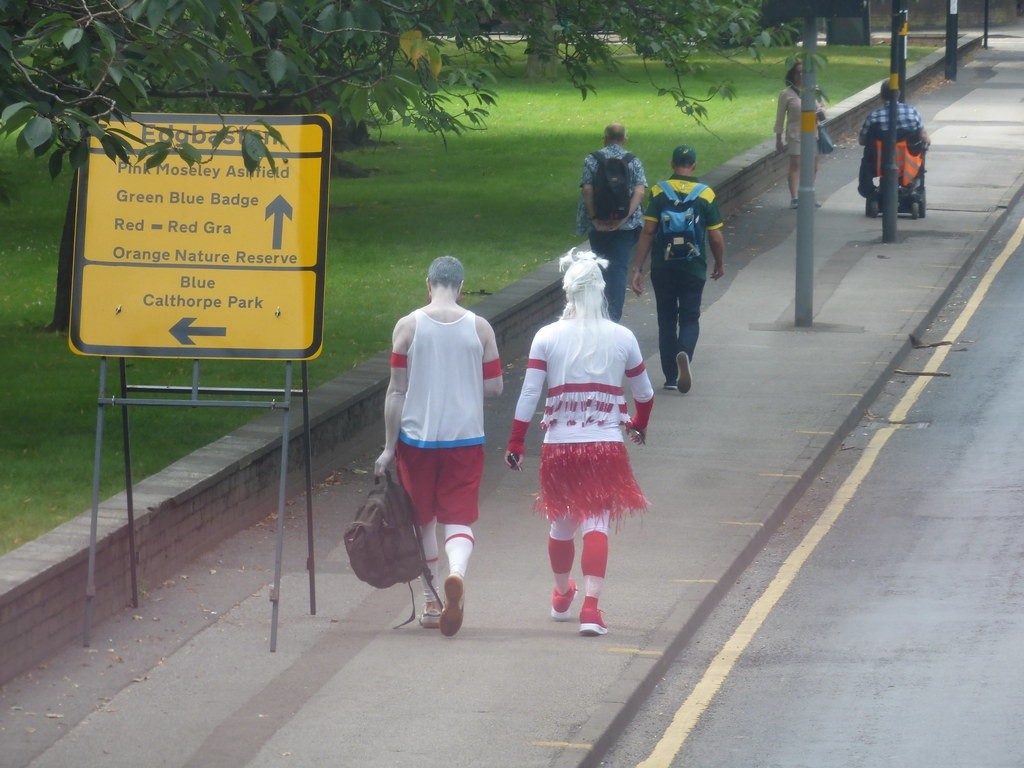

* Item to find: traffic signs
[67,112,334,361]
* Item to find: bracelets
[588,214,596,221]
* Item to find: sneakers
[676,352,691,393]
[551,578,577,620]
[419,609,441,629]
[439,571,465,637]
[664,381,678,389]
[579,608,608,635]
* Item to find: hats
[673,145,696,164]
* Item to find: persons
[574,124,649,321]
[857,79,932,150]
[372,255,505,638]
[773,61,828,209]
[501,247,656,636]
[631,145,724,395]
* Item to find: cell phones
[630,428,646,445]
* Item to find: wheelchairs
[865,122,926,217]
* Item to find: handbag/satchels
[817,126,834,154]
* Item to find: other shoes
[815,199,821,207]
[791,199,798,208]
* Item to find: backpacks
[343,467,444,630]
[589,152,635,220]
[656,182,709,261]
